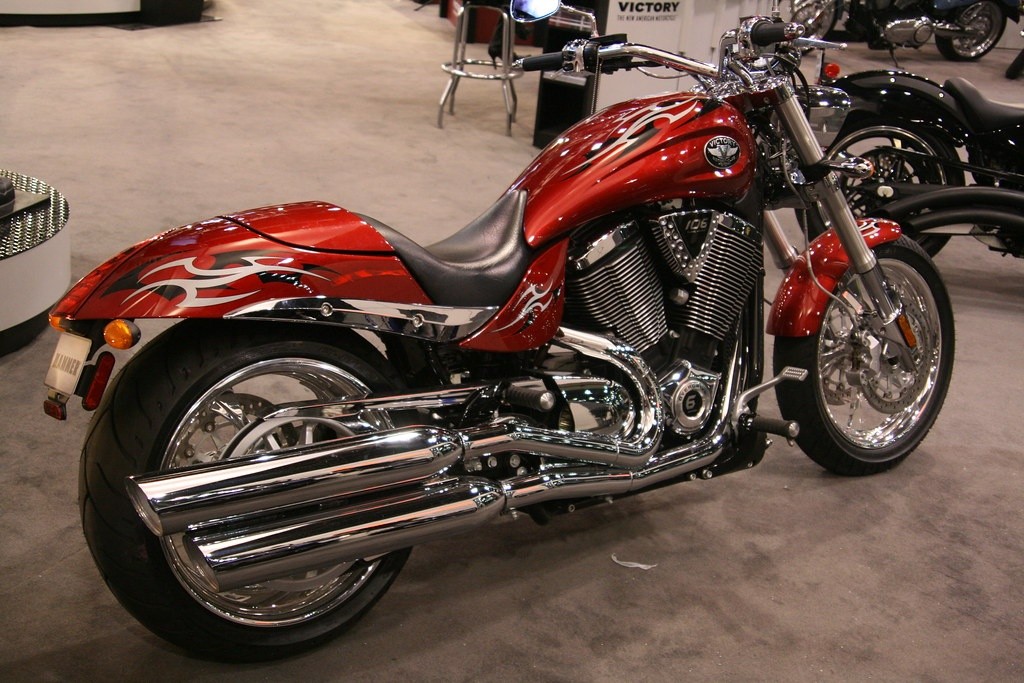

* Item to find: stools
[436,0,524,137]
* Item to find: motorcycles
[797,57,1024,265]
[774,0,1021,63]
[43,0,960,662]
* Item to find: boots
[488,3,532,70]
